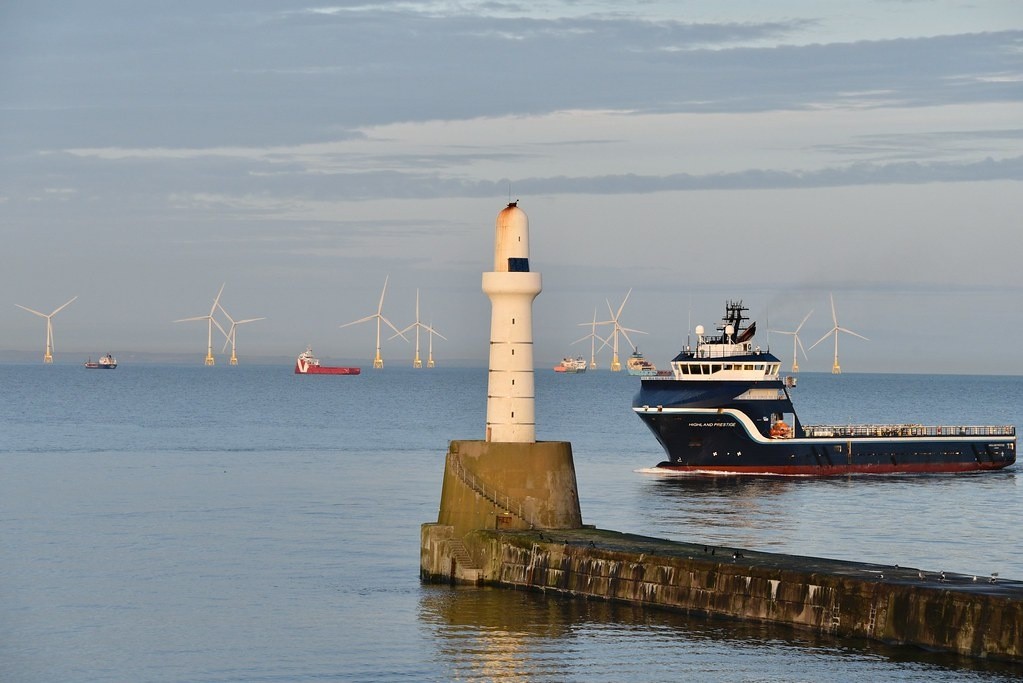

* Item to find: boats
[630,299,1015,477]
[295,344,360,375]
[574,356,587,373]
[554,359,573,372]
[84,352,117,369]
[626,346,658,376]
[657,370,673,376]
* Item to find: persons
[756,344,760,356]
